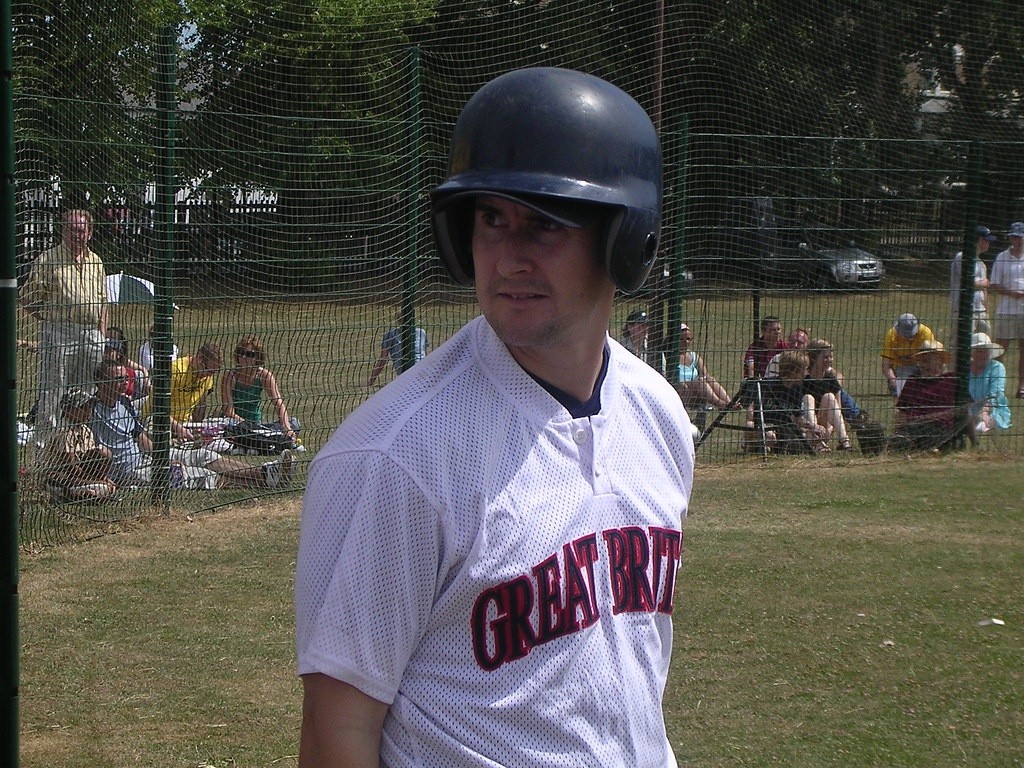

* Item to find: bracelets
[101,319,107,324]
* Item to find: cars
[758,216,887,293]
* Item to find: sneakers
[279,449,294,487]
[262,460,281,488]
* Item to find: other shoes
[837,439,850,449]
[67,484,110,500]
[1016,389,1024,399]
[816,440,832,454]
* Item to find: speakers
[719,195,775,276]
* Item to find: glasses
[235,347,257,359]
[679,337,691,343]
[102,376,129,382]
[203,365,218,376]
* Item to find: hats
[972,227,998,242]
[627,311,650,325]
[60,387,97,411]
[895,313,918,340]
[198,342,226,367]
[912,340,947,357]
[970,333,1005,358]
[1008,223,1024,236]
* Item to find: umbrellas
[104,270,181,310]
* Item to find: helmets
[427,66,661,295]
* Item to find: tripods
[694,276,817,463]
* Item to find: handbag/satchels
[225,417,301,451]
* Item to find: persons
[970,332,1012,438]
[18,209,108,481]
[743,315,885,455]
[295,68,698,768]
[990,222,1024,398]
[950,226,991,373]
[18,327,303,505]
[891,340,975,454]
[620,310,743,411]
[361,311,428,386]
[881,313,935,393]
